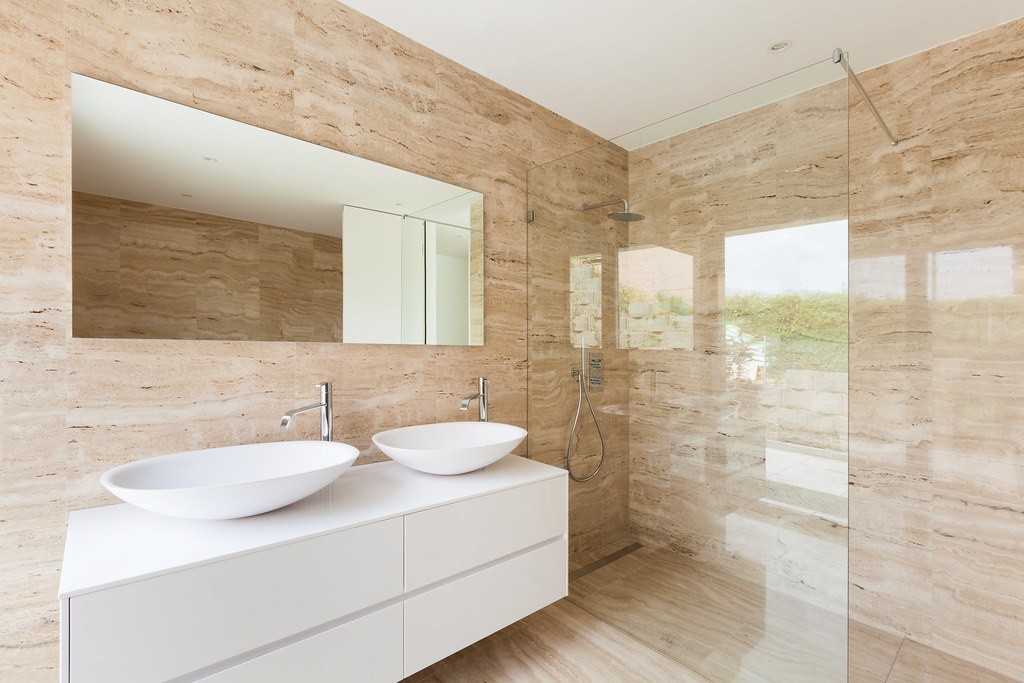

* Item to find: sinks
[99,436,363,523]
[370,420,530,477]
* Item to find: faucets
[458,377,491,421]
[279,381,335,442]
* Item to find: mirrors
[70,71,486,349]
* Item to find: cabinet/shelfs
[59,452,576,683]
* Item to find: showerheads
[607,211,650,225]
